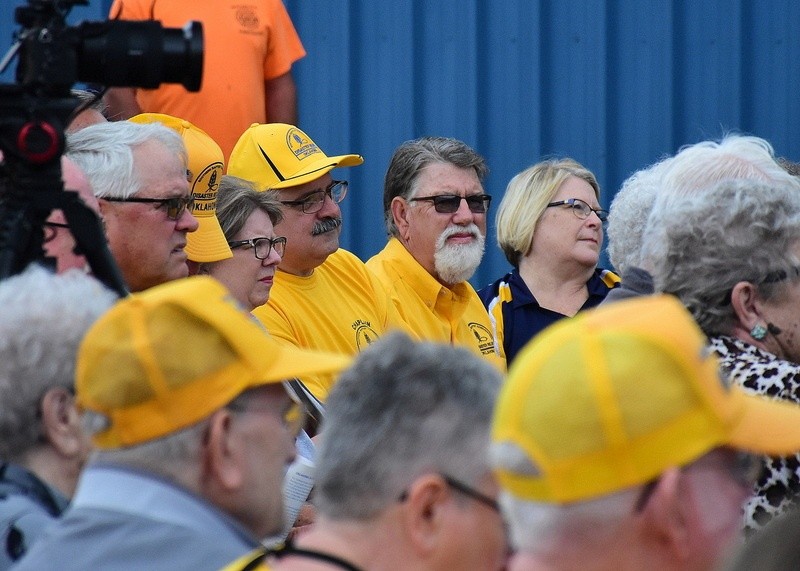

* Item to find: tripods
[1,82,126,298]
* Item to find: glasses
[93,194,197,221]
[227,237,287,260]
[546,198,610,225]
[279,180,348,214]
[408,192,493,213]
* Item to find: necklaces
[268,547,364,571]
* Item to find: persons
[105,0,306,174]
[215,331,519,570]
[606,125,798,546]
[476,147,622,369]
[45,92,235,300]
[187,121,510,384]
[1,261,353,571]
[491,293,799,571]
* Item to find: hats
[123,112,234,265]
[485,290,800,506]
[225,123,364,195]
[73,274,359,450]
